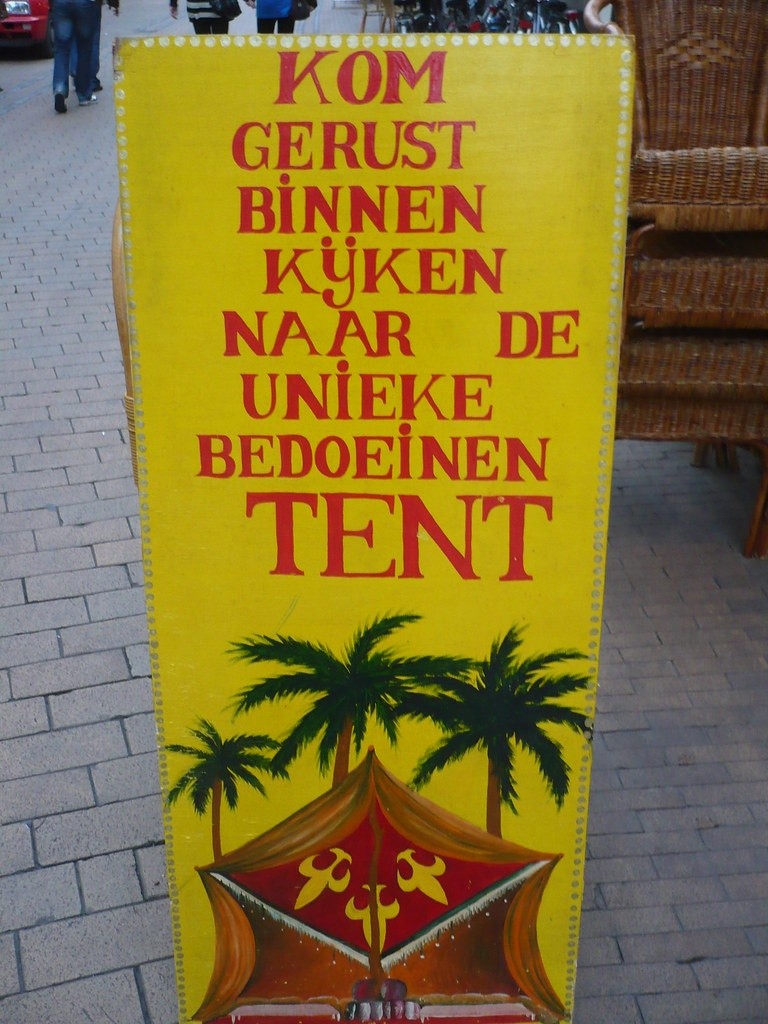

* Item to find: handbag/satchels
[209,0,242,18]
[288,0,317,22]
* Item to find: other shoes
[93,85,103,92]
[79,95,99,105]
[54,94,68,113]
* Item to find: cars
[0,1,57,59]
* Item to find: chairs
[581,0,768,560]
[359,0,402,33]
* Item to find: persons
[250,0,318,35]
[49,0,119,112]
[169,0,254,34]
